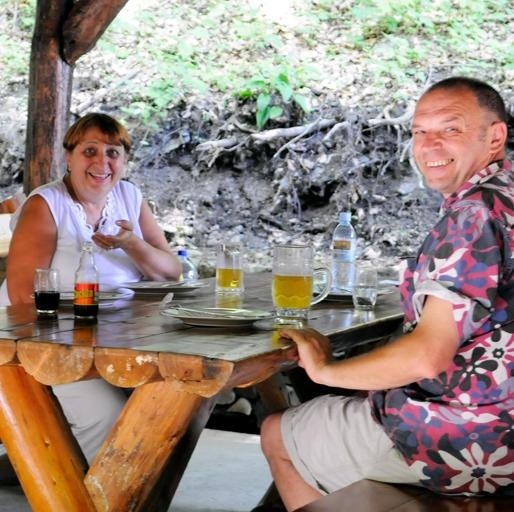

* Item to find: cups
[33,268,60,314]
[350,266,379,311]
[271,243,333,319]
[215,241,244,295]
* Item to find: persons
[257,75,514,512]
[0,111,184,472]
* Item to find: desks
[0,270,417,512]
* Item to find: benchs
[288,479,514,512]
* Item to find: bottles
[72,240,100,321]
[176,249,199,281]
[331,212,357,295]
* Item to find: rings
[106,245,113,250]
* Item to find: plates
[311,281,395,303]
[117,281,210,295]
[159,307,277,328]
[29,285,136,306]
